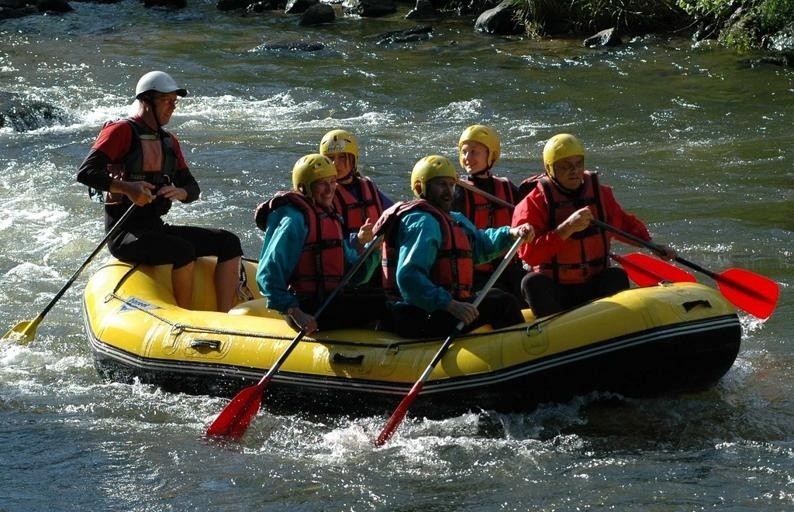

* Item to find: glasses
[154,95,179,105]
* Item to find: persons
[319,130,395,255]
[372,154,526,340]
[256,153,393,334]
[451,124,531,310]
[511,133,678,319]
[77,71,244,313]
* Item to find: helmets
[542,134,585,179]
[292,154,337,188]
[410,155,458,198]
[320,129,360,158]
[133,70,187,97]
[458,124,501,169]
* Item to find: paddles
[591,219,782,318]
[2,200,139,346]
[377,229,532,444]
[208,234,382,442]
[452,176,698,287]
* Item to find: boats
[82,249,740,423]
